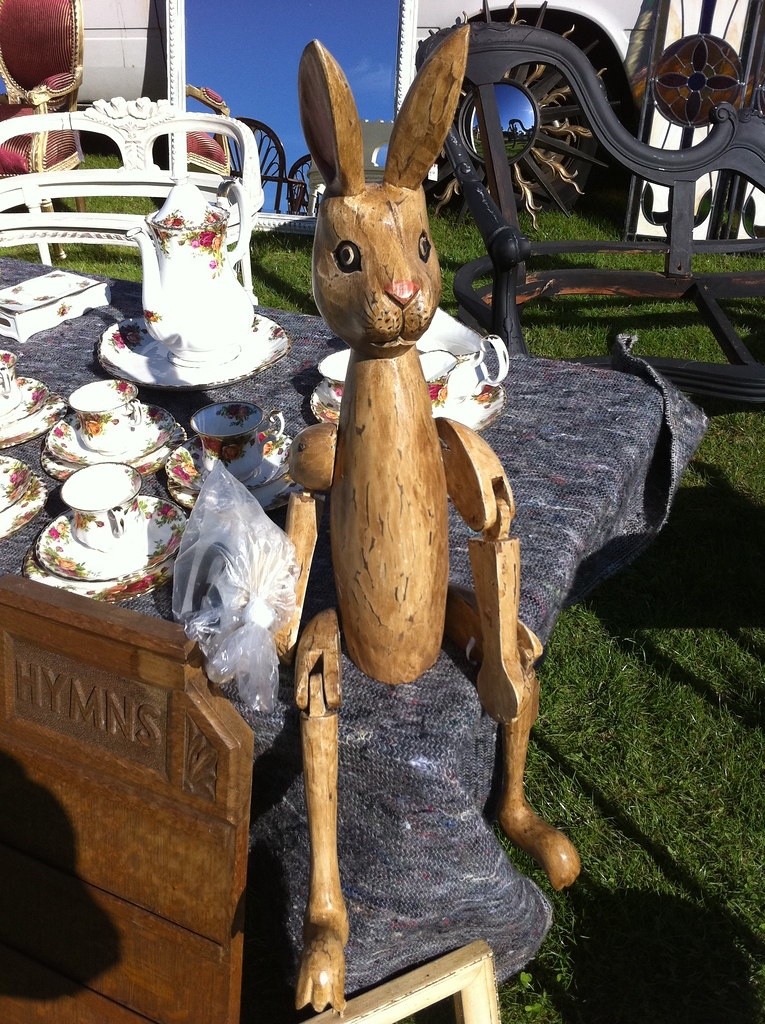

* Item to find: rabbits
[271,22,581,1011]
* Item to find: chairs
[0,0,87,260]
[186,84,230,178]
[0,96,264,305]
[228,116,319,216]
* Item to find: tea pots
[126,173,255,370]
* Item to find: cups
[190,401,285,482]
[59,462,142,551]
[68,379,142,457]
[0,349,23,417]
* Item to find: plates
[97,315,290,391]
[35,495,188,582]
[0,392,68,450]
[45,404,177,465]
[22,541,179,605]
[166,478,304,512]
[0,455,34,514]
[165,434,293,491]
[310,377,505,430]
[0,376,51,428]
[0,472,49,538]
[41,423,187,482]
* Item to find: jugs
[316,332,510,415]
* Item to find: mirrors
[165,0,419,234]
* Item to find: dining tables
[0,256,710,1023]
